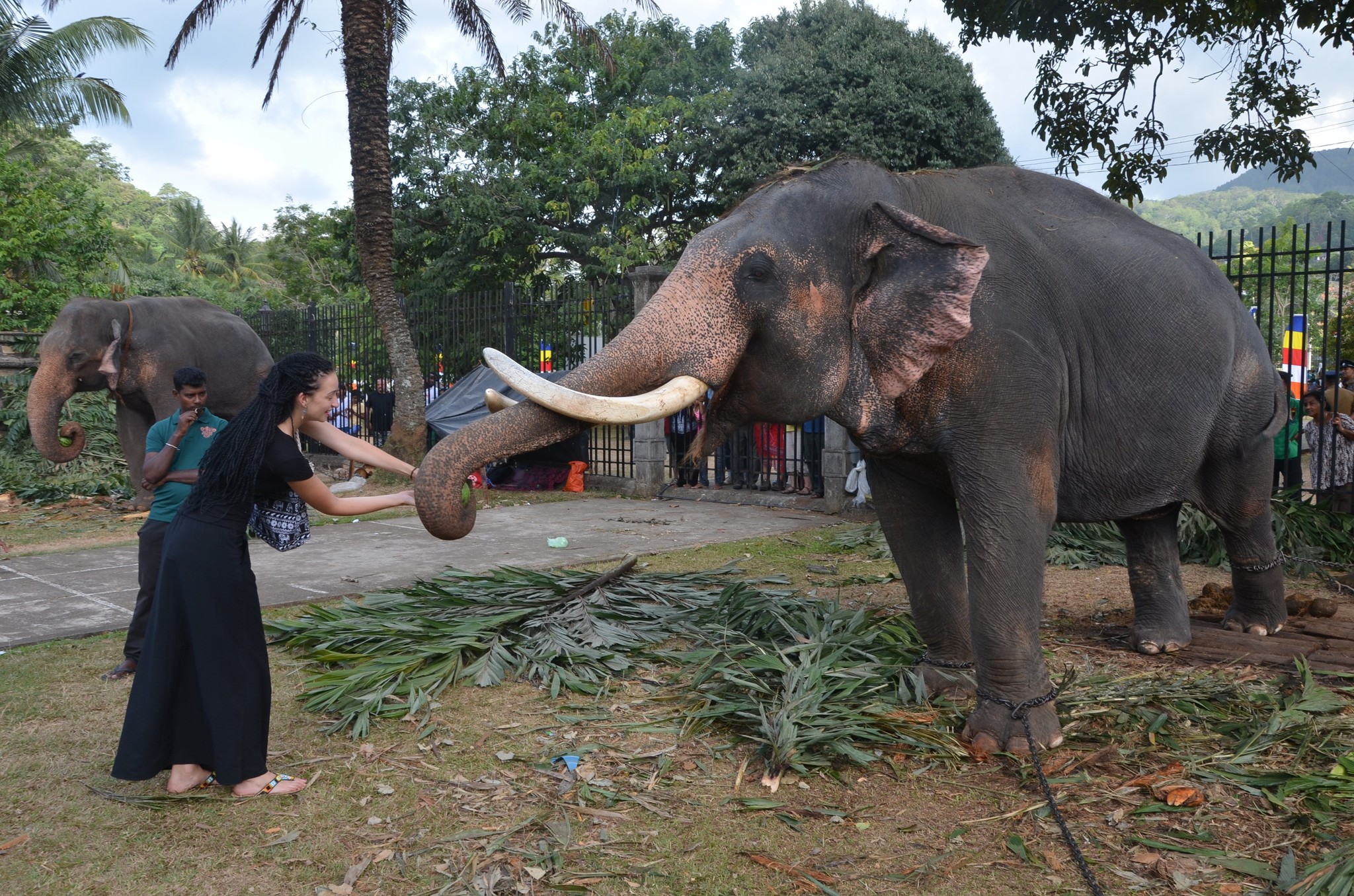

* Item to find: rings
[1336,420,1339,422]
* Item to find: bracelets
[410,467,418,479]
[1341,427,1344,434]
[165,443,181,450]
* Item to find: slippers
[166,770,223,795]
[231,772,307,798]
[101,665,136,681]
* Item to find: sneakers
[759,480,771,491]
[747,481,758,489]
[733,480,744,489]
[771,481,786,491]
[724,477,733,486]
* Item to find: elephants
[414,151,1287,759]
[27,296,275,512]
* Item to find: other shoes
[772,480,777,485]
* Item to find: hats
[1325,371,1343,379]
[1278,371,1292,380]
[1340,359,1354,369]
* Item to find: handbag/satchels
[563,461,587,492]
[247,429,312,552]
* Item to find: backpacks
[487,463,513,484]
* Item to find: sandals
[781,485,799,493]
[811,493,824,499]
[797,487,812,495]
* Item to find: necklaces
[1325,413,1327,419]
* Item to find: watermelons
[461,482,470,507]
[58,436,72,447]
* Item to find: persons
[100,366,228,682]
[1270,359,1354,513]
[328,372,460,449]
[102,351,420,800]
[665,388,825,498]
